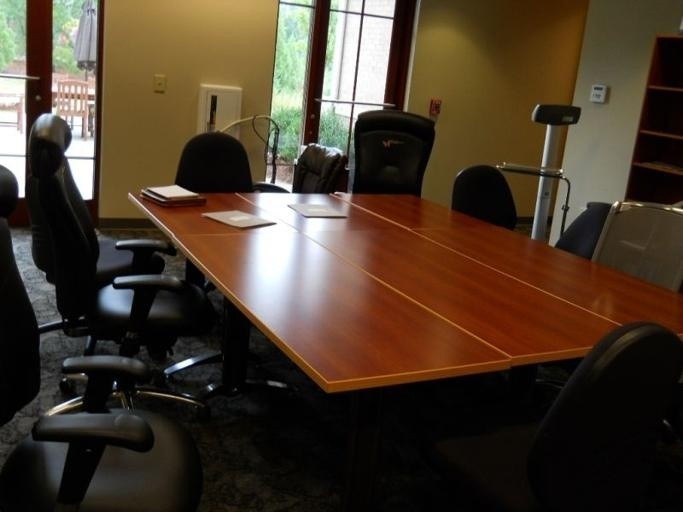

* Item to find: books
[201,210,277,229]
[287,204,348,218]
[139,184,206,208]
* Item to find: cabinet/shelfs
[624,36,682,208]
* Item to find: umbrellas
[74,0,97,81]
